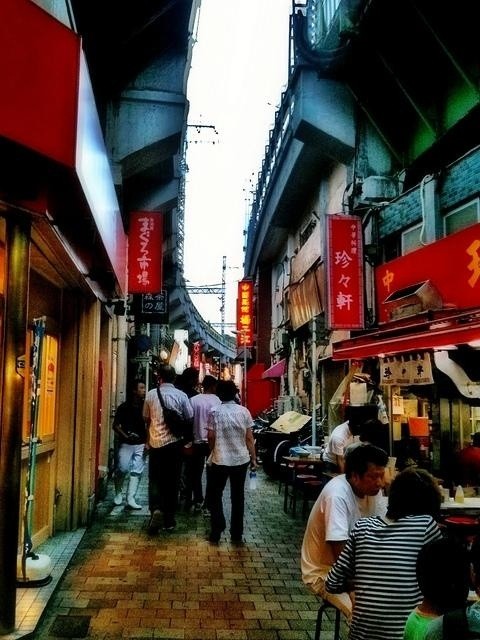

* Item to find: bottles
[249,470,256,490]
[320,446,325,461]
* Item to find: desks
[282,455,323,513]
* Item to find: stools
[279,463,480,640]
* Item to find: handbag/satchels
[162,406,193,438]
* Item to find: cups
[439,489,449,507]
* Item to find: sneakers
[148,508,176,530]
[195,502,212,521]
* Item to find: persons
[324,470,444,639]
[403,531,480,639]
[301,445,389,626]
[460,432,479,464]
[111,368,258,545]
[395,438,421,472]
[323,412,374,473]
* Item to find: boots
[114,479,122,505]
[127,476,143,509]
